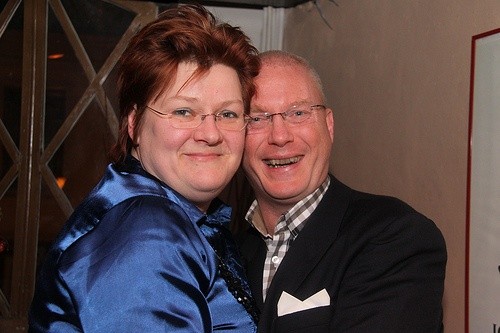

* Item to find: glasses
[145,104,251,131]
[250,103,326,130]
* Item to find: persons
[37,9,260,332]
[223,50,448,333]
